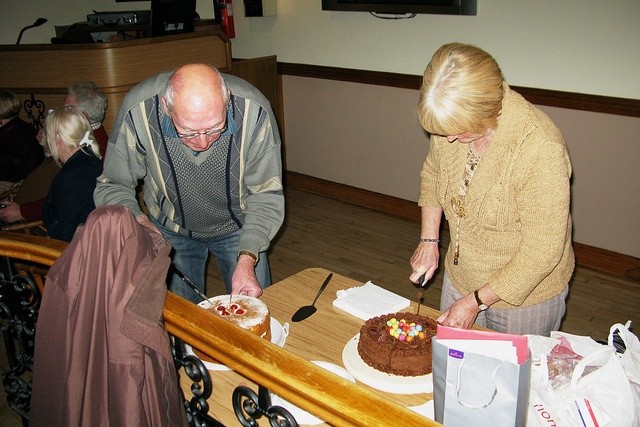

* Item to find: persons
[410,42,576,337]
[0,81,109,224]
[40,103,104,243]
[0,87,46,194]
[91,63,285,305]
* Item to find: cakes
[193,295,272,365]
[360,311,443,376]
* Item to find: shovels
[291,273,333,322]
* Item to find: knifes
[412,280,430,328]
[164,256,214,306]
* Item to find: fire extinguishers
[218,0,235,39]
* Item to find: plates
[270,359,356,425]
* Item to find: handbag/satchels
[432,336,532,427]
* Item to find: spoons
[291,274,336,327]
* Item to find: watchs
[474,290,489,311]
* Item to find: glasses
[170,114,229,139]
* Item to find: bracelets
[419,239,439,243]
[237,251,256,262]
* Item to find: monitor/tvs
[321,0,477,16]
[61,30,95,44]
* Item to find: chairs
[0,186,47,236]
